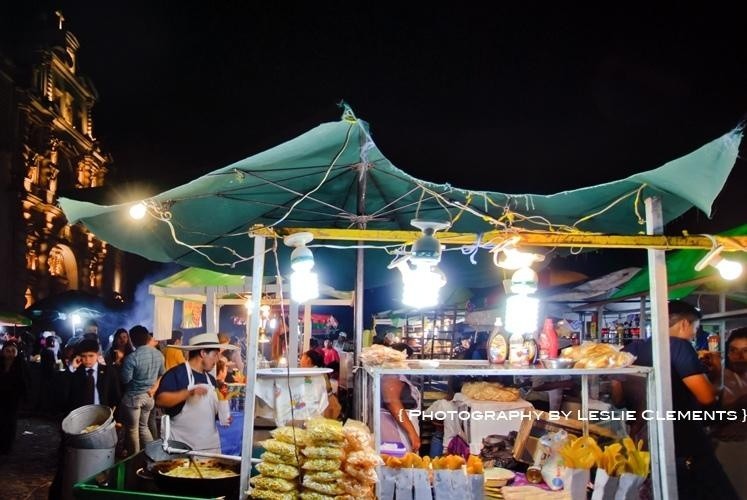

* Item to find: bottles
[527,435,552,485]
[508,329,524,364]
[705,329,719,355]
[602,320,641,345]
[539,318,560,360]
[523,333,540,365]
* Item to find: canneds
[602,327,625,344]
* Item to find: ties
[87,369,94,404]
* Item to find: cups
[216,399,231,427]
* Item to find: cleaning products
[536,318,558,361]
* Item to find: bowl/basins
[535,357,577,369]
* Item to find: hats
[167,333,239,350]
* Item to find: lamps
[502,265,540,334]
[389,255,413,306]
[402,215,452,310]
[128,199,147,220]
[281,229,325,306]
[690,243,744,281]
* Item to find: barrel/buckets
[145,438,195,466]
[63,447,116,488]
[216,412,244,454]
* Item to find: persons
[610,300,717,500]
[1,325,244,458]
[720,327,747,405]
[295,329,488,457]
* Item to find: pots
[145,457,254,484]
